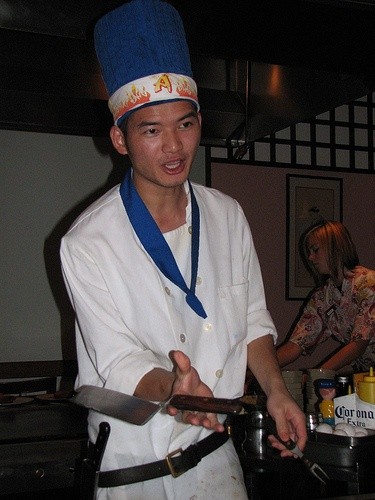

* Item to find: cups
[305,413,323,432]
[244,407,270,456]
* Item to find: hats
[93,1,204,121]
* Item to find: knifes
[84,422,111,471]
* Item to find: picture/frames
[285,174,343,301]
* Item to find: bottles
[359,367,375,405]
[337,377,351,397]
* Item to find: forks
[263,416,331,486]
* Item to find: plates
[0,396,34,405]
[35,394,78,400]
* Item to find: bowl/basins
[306,368,335,412]
[238,393,268,411]
[281,370,304,411]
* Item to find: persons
[59,0,307,500]
[245,220,375,394]
